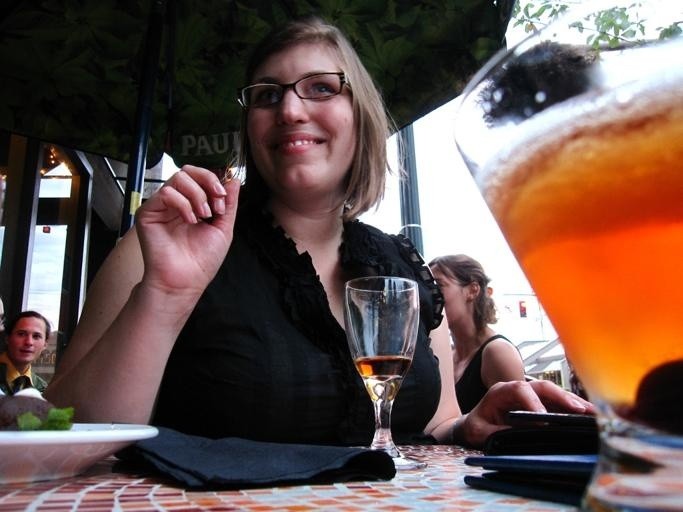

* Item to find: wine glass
[343,276,420,470]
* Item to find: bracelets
[447,419,461,445]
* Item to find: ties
[12,375,28,393]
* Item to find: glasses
[230,71,352,113]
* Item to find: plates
[1,421,160,486]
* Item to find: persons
[0,310,49,399]
[43,12,601,450]
[427,253,526,414]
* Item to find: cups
[451,1,683,512]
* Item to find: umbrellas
[0,0,517,254]
